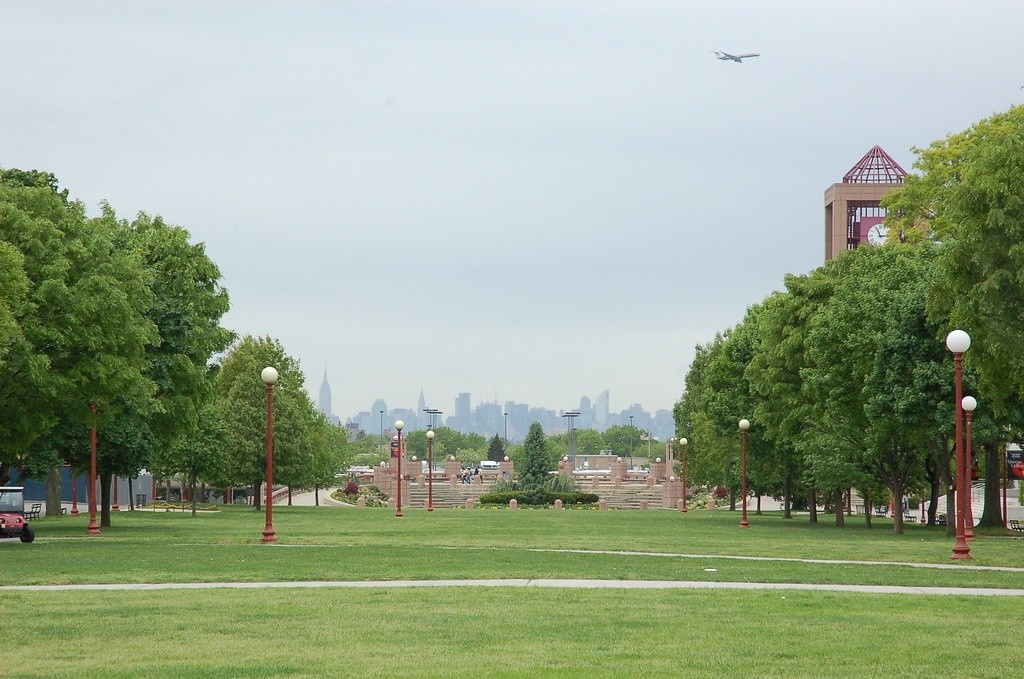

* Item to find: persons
[460,466,484,485]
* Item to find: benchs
[1010,519,1024,533]
[24,503,42,521]
[934,514,946,525]
[875,507,886,517]
[903,509,917,523]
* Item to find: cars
[0,486,35,543]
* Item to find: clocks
[866,223,891,247]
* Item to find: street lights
[504,412,508,456]
[260,365,279,543]
[395,420,405,517]
[739,418,751,529]
[680,437,688,512]
[945,330,973,560]
[379,410,384,462]
[629,416,633,470]
[426,430,436,512]
[961,396,977,542]
